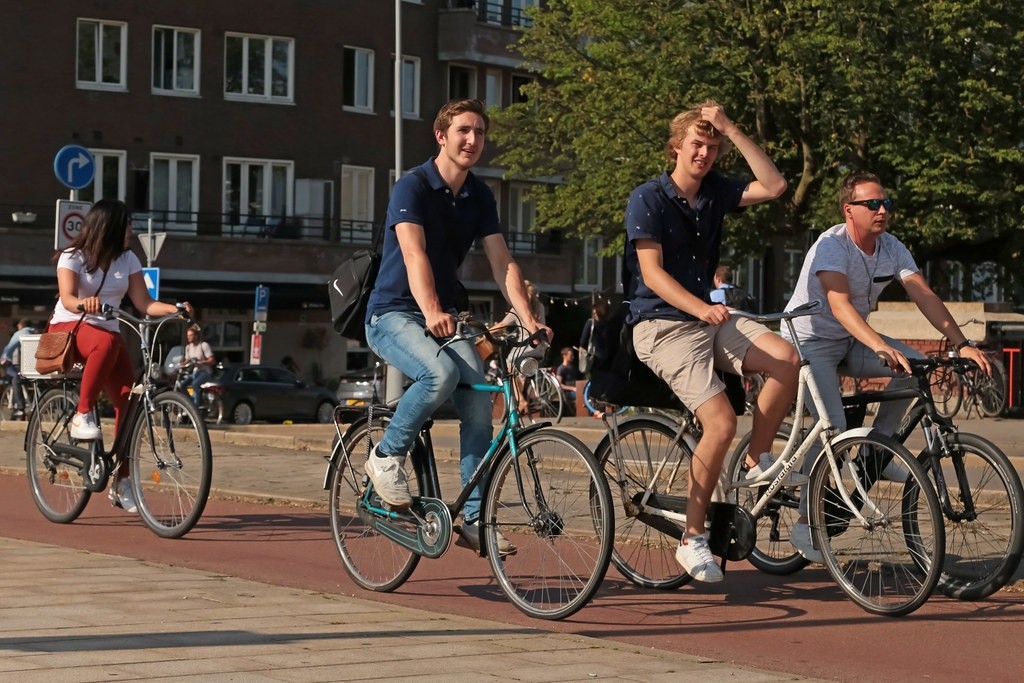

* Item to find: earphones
[847,209,853,214]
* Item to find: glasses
[848,199,892,210]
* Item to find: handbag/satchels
[327,248,379,342]
[34,332,78,375]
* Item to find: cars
[170,364,343,426]
[336,365,387,417]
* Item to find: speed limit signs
[56,199,94,249]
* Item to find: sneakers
[789,521,826,564]
[675,531,724,583]
[70,410,104,440]
[881,460,909,483]
[108,471,139,513]
[455,518,518,558]
[364,441,413,508]
[740,452,809,488]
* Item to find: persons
[781,173,992,562]
[50,198,194,513]
[0,267,736,420]
[623,99,800,581]
[366,98,551,553]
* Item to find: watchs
[955,338,976,353]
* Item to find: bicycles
[590,298,947,619]
[477,326,564,434]
[725,349,1024,602]
[23,302,211,539]
[322,311,616,621]
[922,318,1009,421]
[0,356,63,423]
